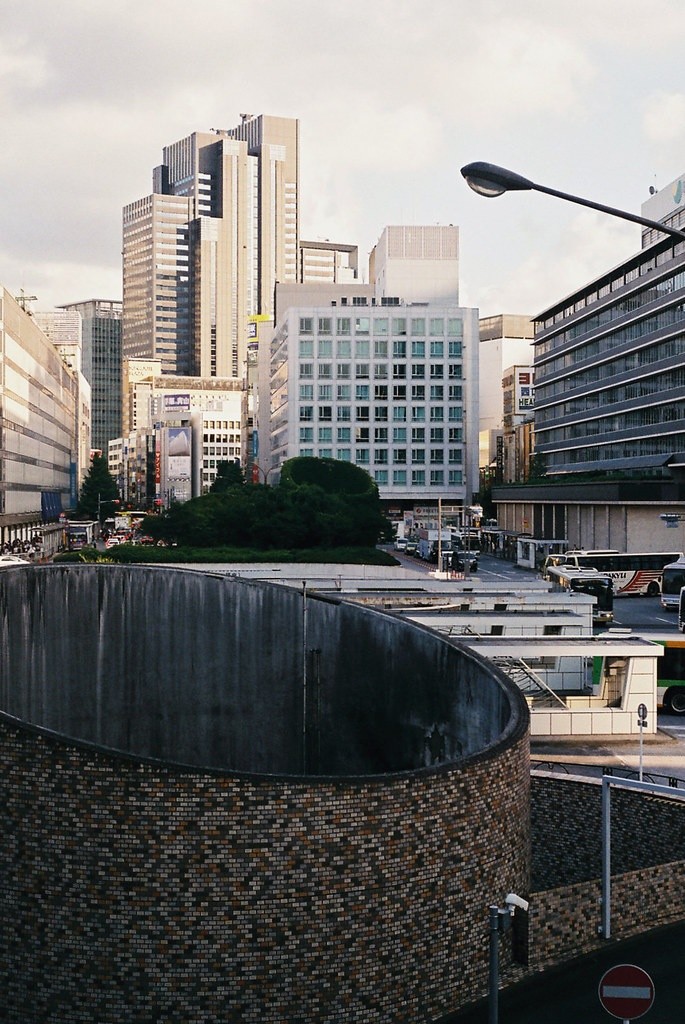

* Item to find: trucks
[417,528,452,564]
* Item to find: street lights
[234,457,285,486]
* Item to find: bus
[661,556,685,609]
[592,630,685,716]
[545,564,614,627]
[677,585,685,635]
[543,550,685,599]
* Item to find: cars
[405,542,419,556]
[416,545,421,557]
[441,550,455,567]
[457,552,478,572]
[394,537,409,552]
[105,515,169,550]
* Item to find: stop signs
[598,964,655,1020]
